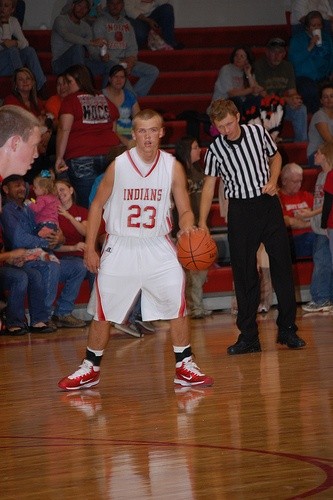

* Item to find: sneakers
[58,359,101,392]
[174,354,214,387]
[227,339,261,355]
[276,330,306,348]
[302,300,333,312]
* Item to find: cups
[99,44,107,56]
[312,29,322,45]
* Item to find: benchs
[0,24,322,311]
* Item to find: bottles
[23,198,36,207]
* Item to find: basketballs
[177,231,217,272]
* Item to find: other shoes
[50,254,60,265]
[37,88,49,100]
[256,304,269,314]
[33,248,50,263]
[204,309,213,315]
[51,313,87,327]
[161,38,184,51]
[4,327,27,336]
[133,316,154,335]
[113,321,144,338]
[47,320,57,329]
[190,310,203,318]
[232,308,238,317]
[29,323,52,334]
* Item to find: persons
[0,105,41,181]
[198,97,306,354]
[58,109,214,391]
[0,0,333,336]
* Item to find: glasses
[267,41,287,49]
